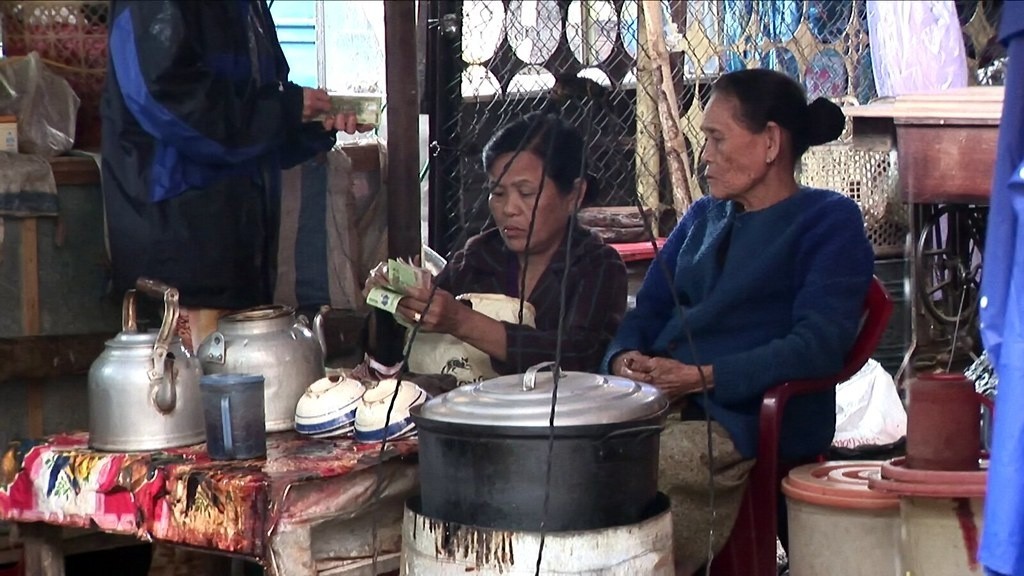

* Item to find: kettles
[86,277,206,451]
[196,305,332,433]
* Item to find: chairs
[701,273,896,576]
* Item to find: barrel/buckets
[868,456,989,575]
[781,460,901,576]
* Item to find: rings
[413,312,424,322]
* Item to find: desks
[1,430,421,576]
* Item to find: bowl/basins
[295,375,434,445]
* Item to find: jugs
[905,372,994,471]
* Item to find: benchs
[608,237,670,263]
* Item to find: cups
[200,372,267,461]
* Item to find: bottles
[0,115,18,154]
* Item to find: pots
[410,361,670,532]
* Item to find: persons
[102,0,377,330]
[362,110,628,388]
[597,69,876,575]
